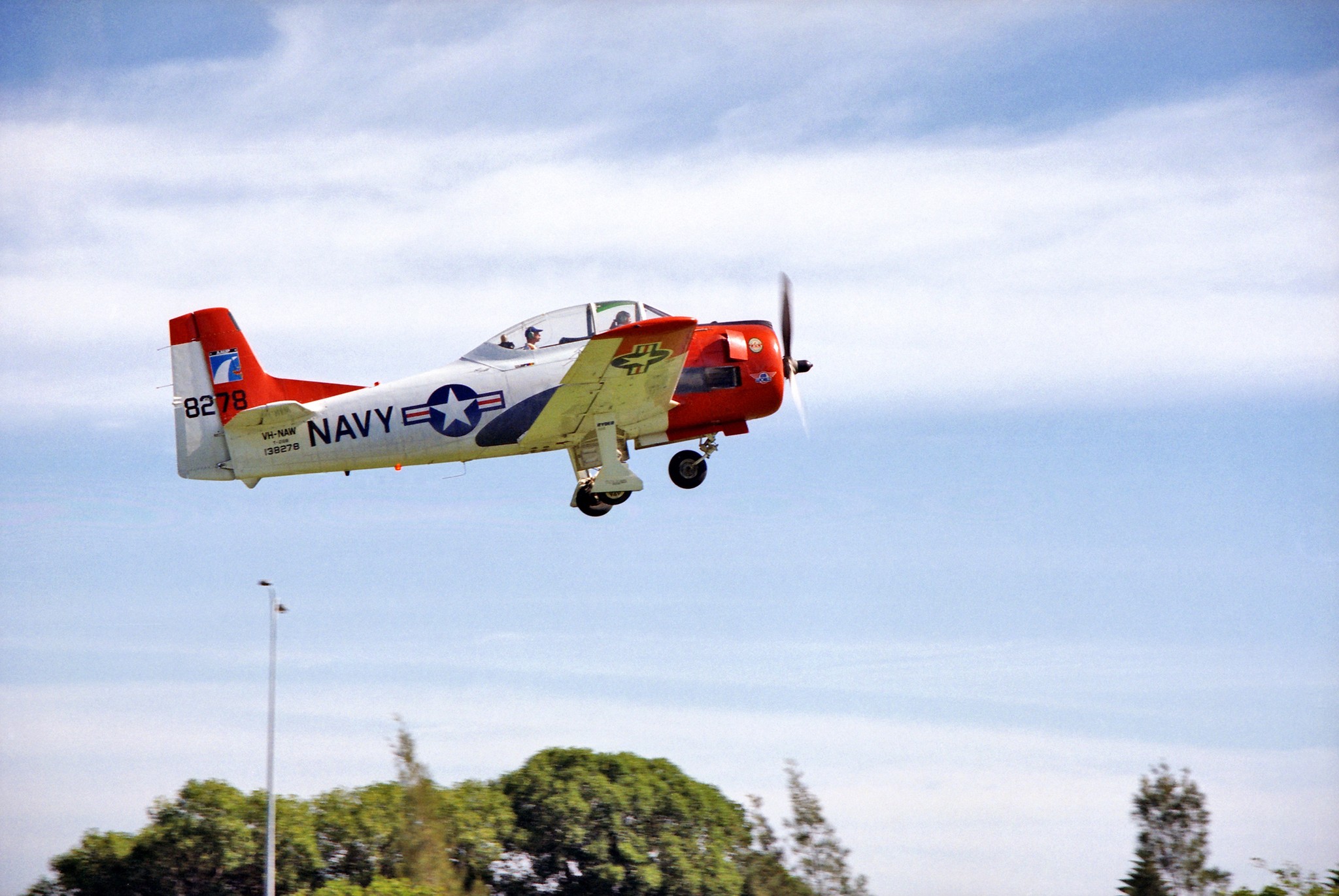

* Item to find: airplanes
[170,269,812,518]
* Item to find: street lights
[258,578,287,896]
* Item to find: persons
[610,311,632,330]
[498,334,515,349]
[520,326,543,366]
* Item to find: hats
[525,327,543,337]
